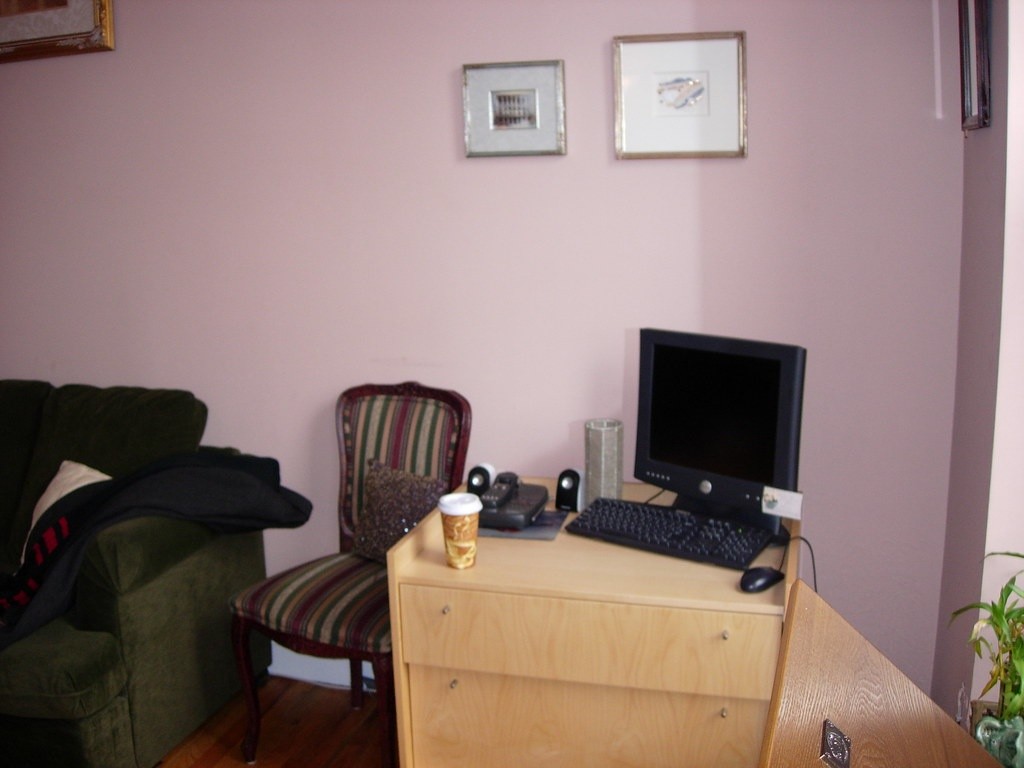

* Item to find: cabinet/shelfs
[386,475,802,768]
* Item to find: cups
[438,492,483,570]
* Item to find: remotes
[481,482,511,506]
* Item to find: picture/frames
[462,59,568,158]
[0,0,115,63]
[609,30,746,160]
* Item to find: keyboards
[566,497,776,572]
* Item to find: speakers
[555,466,585,514]
[468,464,495,498]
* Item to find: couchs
[0,380,265,768]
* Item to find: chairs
[231,381,473,768]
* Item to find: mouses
[741,567,785,592]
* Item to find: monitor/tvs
[635,328,807,544]
